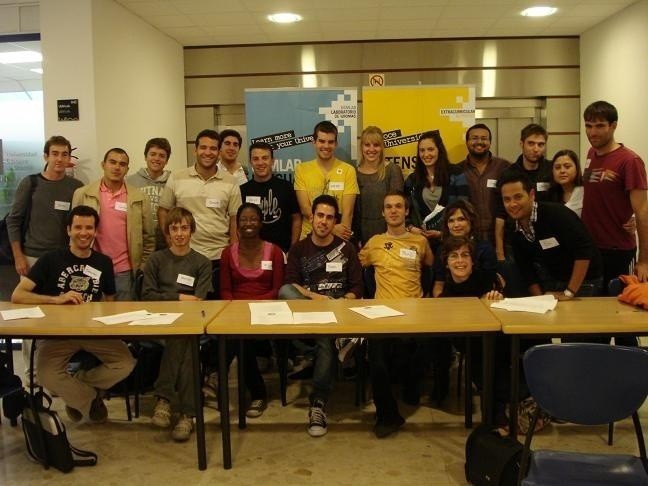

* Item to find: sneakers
[152,400,171,424]
[374,410,406,438]
[91,398,108,423]
[306,404,329,437]
[173,414,195,440]
[247,398,263,415]
[202,370,219,396]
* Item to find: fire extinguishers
[43,147,79,178]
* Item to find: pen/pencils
[201,310,206,317]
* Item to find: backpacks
[0,173,37,262]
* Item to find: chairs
[518,343,648,486]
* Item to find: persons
[5,100,647,443]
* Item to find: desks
[481,295,648,439]
[0,301,231,470]
[206,297,502,470]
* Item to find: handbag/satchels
[19,390,97,474]
[462,425,534,485]
